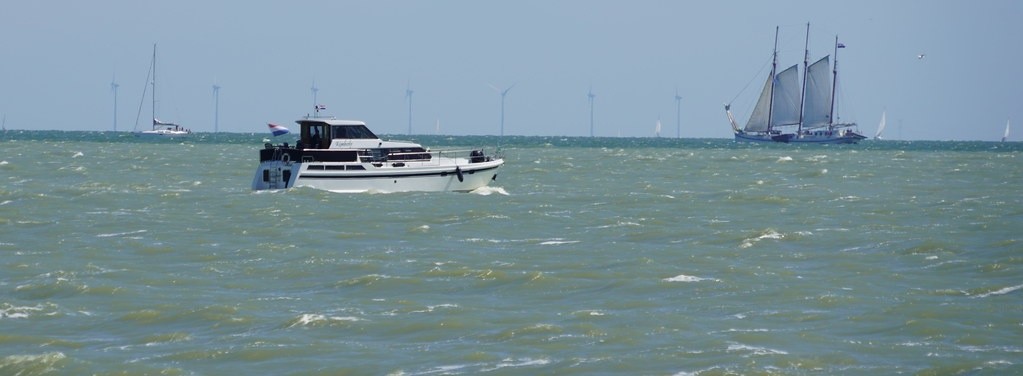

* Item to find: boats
[250,82,505,194]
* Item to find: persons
[313,130,321,148]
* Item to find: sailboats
[722,20,869,146]
[125,38,194,137]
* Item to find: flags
[268,124,291,137]
